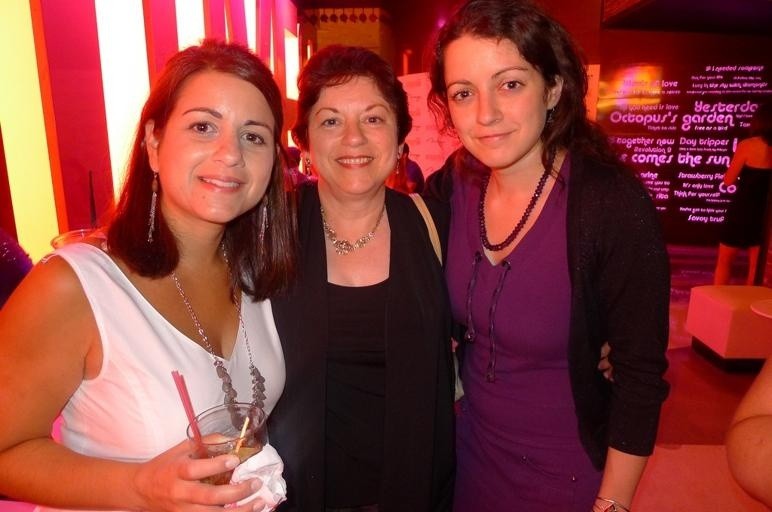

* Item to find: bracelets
[593,495,624,511]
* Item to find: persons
[253,44,620,510]
[420,0,674,512]
[712,98,771,287]
[724,355,772,511]
[1,37,287,512]
[279,146,311,193]
[401,144,426,195]
[387,160,416,194]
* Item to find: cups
[186,401,268,485]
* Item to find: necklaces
[172,239,266,432]
[320,199,385,259]
[477,148,559,252]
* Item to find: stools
[685,285,772,373]
